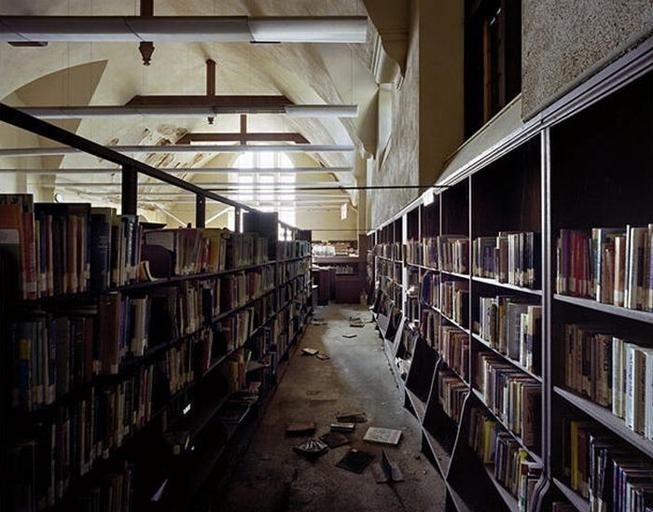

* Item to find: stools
[335,276,362,304]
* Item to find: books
[366,224,652,512]
[301,316,365,360]
[311,241,355,273]
[2,193,316,511]
[284,411,405,484]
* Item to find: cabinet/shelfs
[0,102,312,512]
[367,37,653,512]
